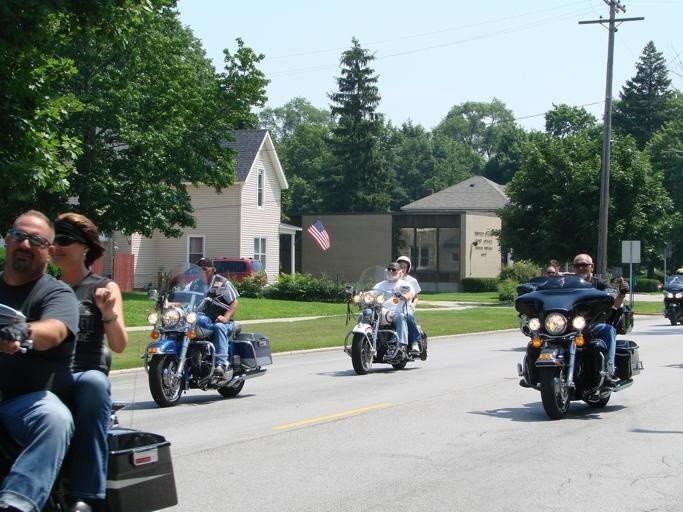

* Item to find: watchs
[101,314,119,325]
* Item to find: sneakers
[344,343,352,354]
[412,342,420,352]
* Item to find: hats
[195,257,216,267]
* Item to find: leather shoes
[214,363,225,377]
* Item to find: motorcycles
[1,303,178,510]
[514,275,643,417]
[344,266,427,374]
[141,265,272,406]
[657,275,682,326]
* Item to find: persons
[370,262,411,357]
[546,267,556,277]
[565,255,629,383]
[0,210,79,507]
[50,212,127,508]
[187,258,240,373]
[394,256,421,353]
[549,259,561,276]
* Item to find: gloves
[617,279,631,297]
[1,320,32,345]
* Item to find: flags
[307,218,331,253]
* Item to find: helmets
[395,255,411,274]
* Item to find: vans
[170,259,265,294]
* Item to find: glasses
[53,234,86,247]
[546,271,556,274]
[387,267,397,272]
[201,267,209,271]
[6,228,52,247]
[574,263,592,267]
